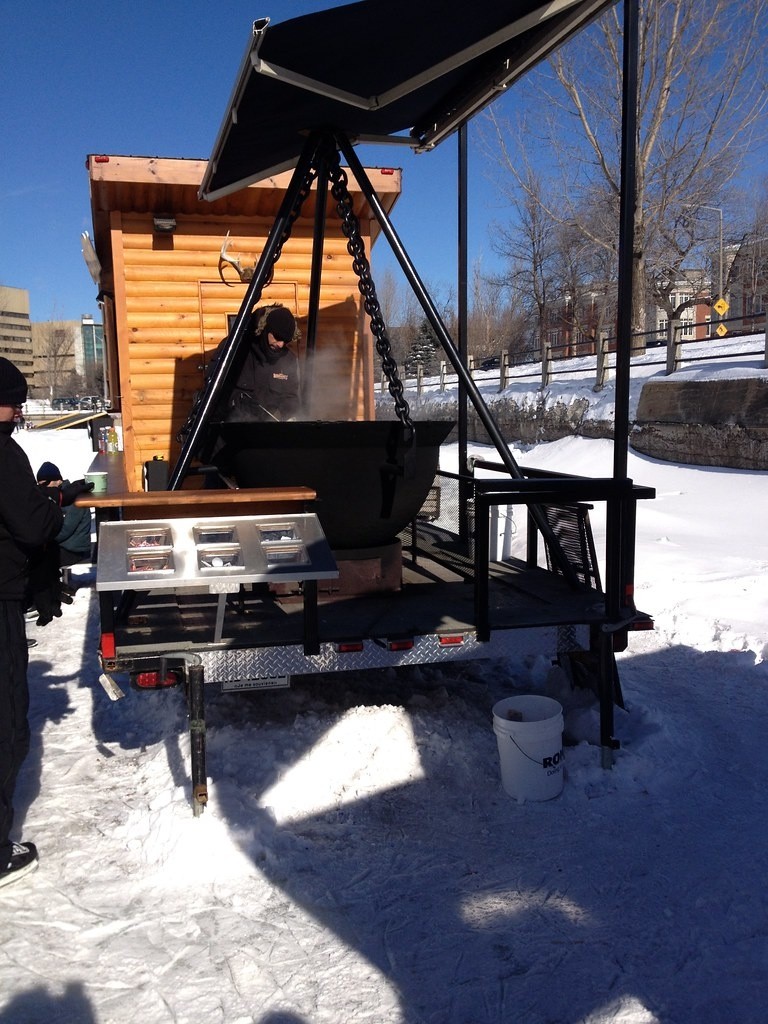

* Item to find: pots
[203,420,454,544]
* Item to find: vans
[52,398,82,410]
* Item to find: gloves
[35,576,75,626]
[37,479,95,508]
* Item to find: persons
[26,607,39,649]
[14,416,34,431]
[36,460,93,625]
[213,302,301,423]
[0,354,96,890]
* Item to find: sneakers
[26,638,37,648]
[0,840,39,890]
[25,607,40,624]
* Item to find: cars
[81,396,110,408]
[480,356,502,368]
[646,339,669,347]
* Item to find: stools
[60,542,98,586]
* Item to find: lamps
[154,214,176,234]
[96,289,114,306]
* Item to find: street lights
[680,202,725,338]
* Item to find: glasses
[9,404,23,422]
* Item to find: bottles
[98,426,118,456]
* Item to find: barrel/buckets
[494,696,565,802]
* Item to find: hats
[0,357,29,405]
[265,308,295,344]
[37,462,63,482]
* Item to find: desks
[74,488,318,650]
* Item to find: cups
[83,472,108,492]
